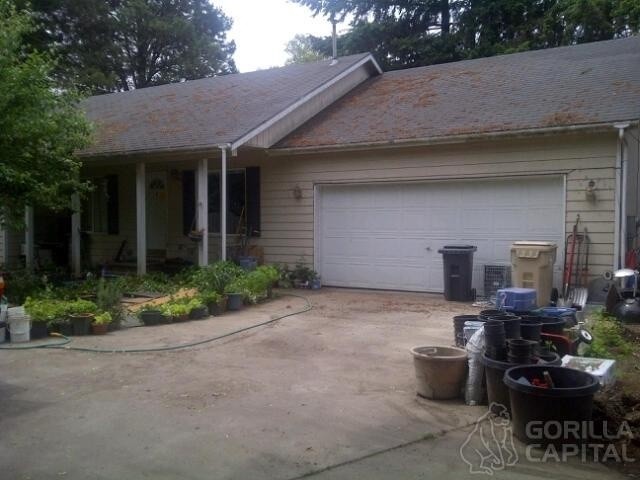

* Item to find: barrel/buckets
[478,342,562,419]
[0,306,33,344]
[503,365,600,449]
[250,246,265,260]
[410,346,469,399]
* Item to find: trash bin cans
[240,256,256,272]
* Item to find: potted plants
[23,252,323,343]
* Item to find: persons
[631,224,640,268]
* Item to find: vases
[410,310,600,450]
